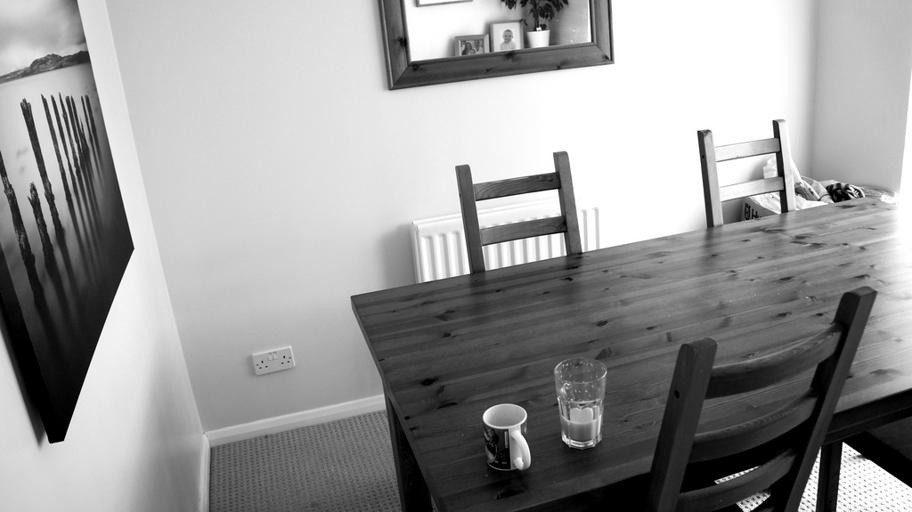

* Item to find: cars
[481,402,532,476]
[553,355,607,450]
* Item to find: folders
[409,194,601,283]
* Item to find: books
[740,196,778,222]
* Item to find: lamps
[588,285,878,511]
[455,151,582,274]
[697,118,793,229]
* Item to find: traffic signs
[251,346,296,376]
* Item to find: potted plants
[489,21,525,52]
[455,34,489,57]
[416,0,473,7]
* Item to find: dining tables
[500,0,568,48]
